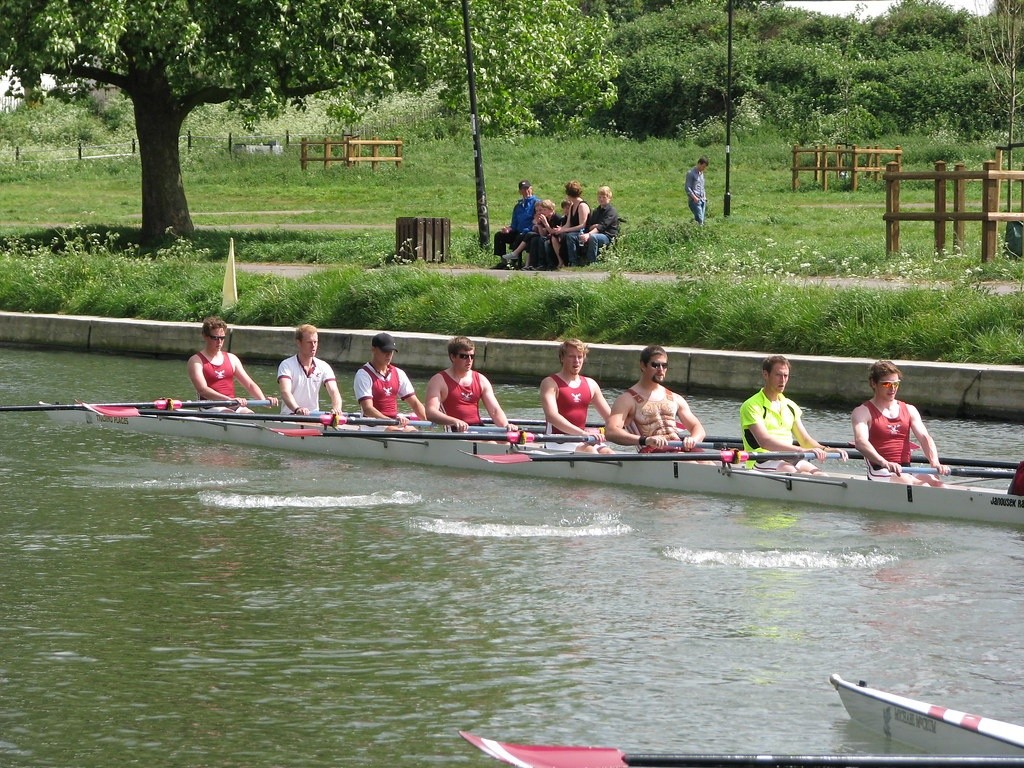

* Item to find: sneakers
[502,252,519,260]
[521,266,531,271]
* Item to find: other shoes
[529,264,548,271]
[493,262,506,269]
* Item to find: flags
[221,241,237,305]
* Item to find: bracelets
[294,407,300,414]
[823,446,830,450]
[638,435,647,445]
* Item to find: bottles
[579,229,584,246]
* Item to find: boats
[827,672,1024,758]
[34,395,1024,529]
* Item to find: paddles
[655,439,1020,470]
[457,729,1024,768]
[0,400,271,413]
[253,421,598,447]
[678,433,921,450]
[889,467,1017,479]
[458,447,843,464]
[80,402,434,430]
[289,410,421,421]
[399,411,687,432]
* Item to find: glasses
[206,332,225,340]
[652,362,668,368]
[451,352,474,359]
[880,380,901,388]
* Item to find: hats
[372,333,398,352]
[519,181,531,189]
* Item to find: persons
[852,362,951,487]
[539,338,614,454]
[353,333,426,432]
[491,180,619,271]
[425,337,518,444]
[685,157,709,225]
[605,345,716,466]
[187,317,279,414]
[276,324,342,427]
[740,357,849,477]
